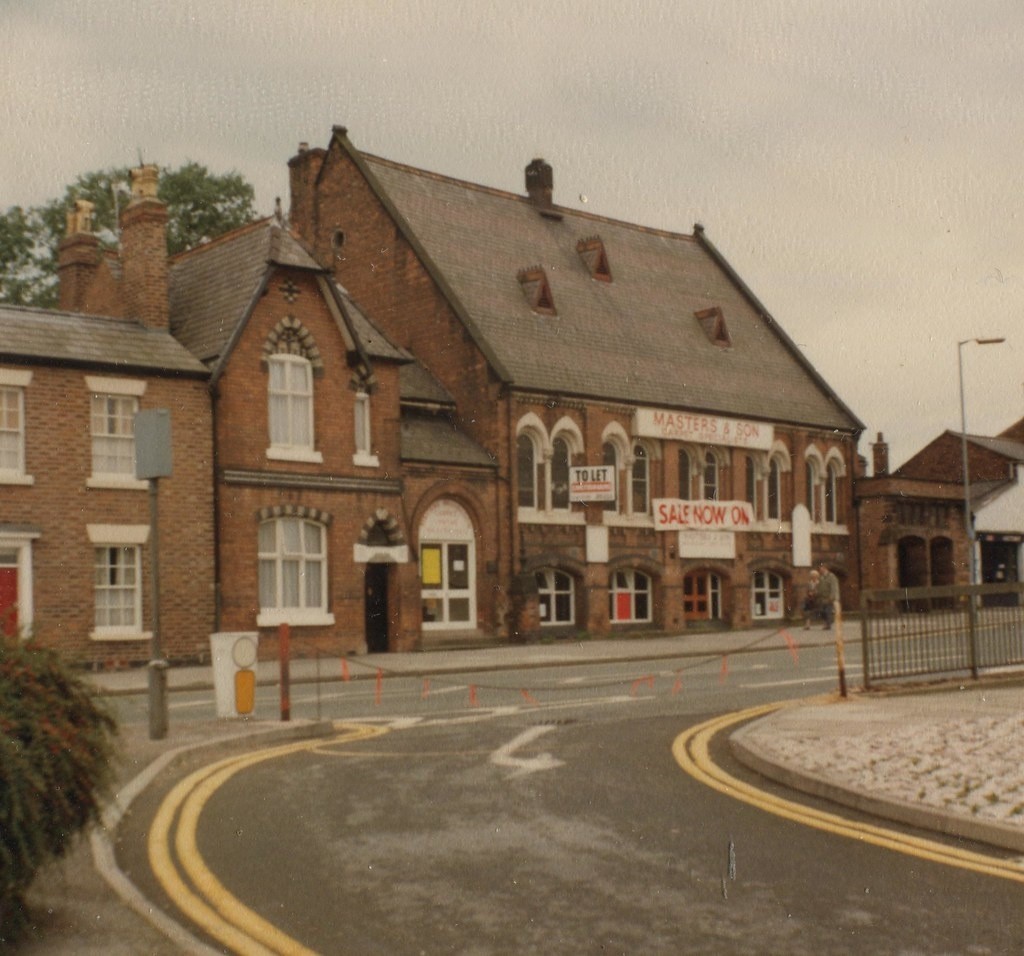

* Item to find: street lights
[957,335,1007,679]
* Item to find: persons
[803,562,838,630]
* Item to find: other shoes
[824,626,831,630]
